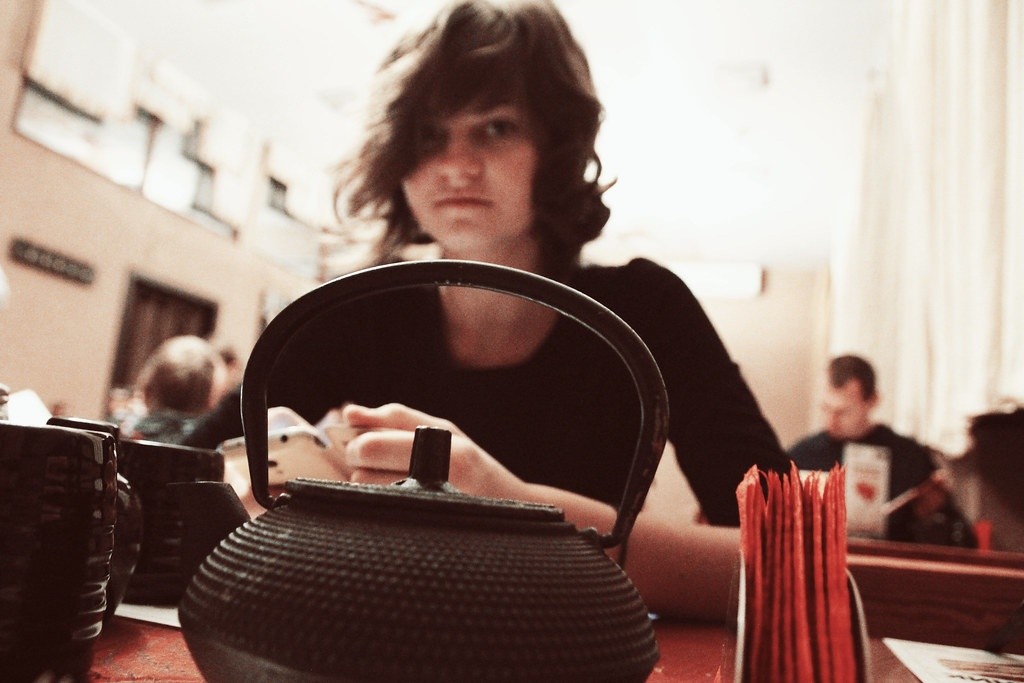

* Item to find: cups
[117,438,224,606]
[0,422,116,683]
[123,482,251,605]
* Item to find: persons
[125,336,228,446]
[182,0,838,626]
[787,355,979,548]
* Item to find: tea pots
[167,260,669,683]
